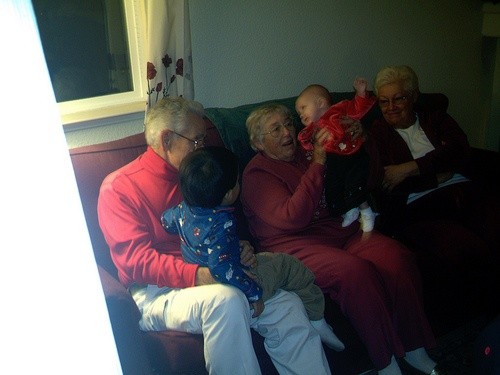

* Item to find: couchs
[68,92,500,375]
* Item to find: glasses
[172,131,207,150]
[261,119,293,137]
[377,93,408,106]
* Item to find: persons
[239,104,443,375]
[97,96,331,375]
[294,79,376,232]
[161,146,345,352]
[365,66,500,290]
